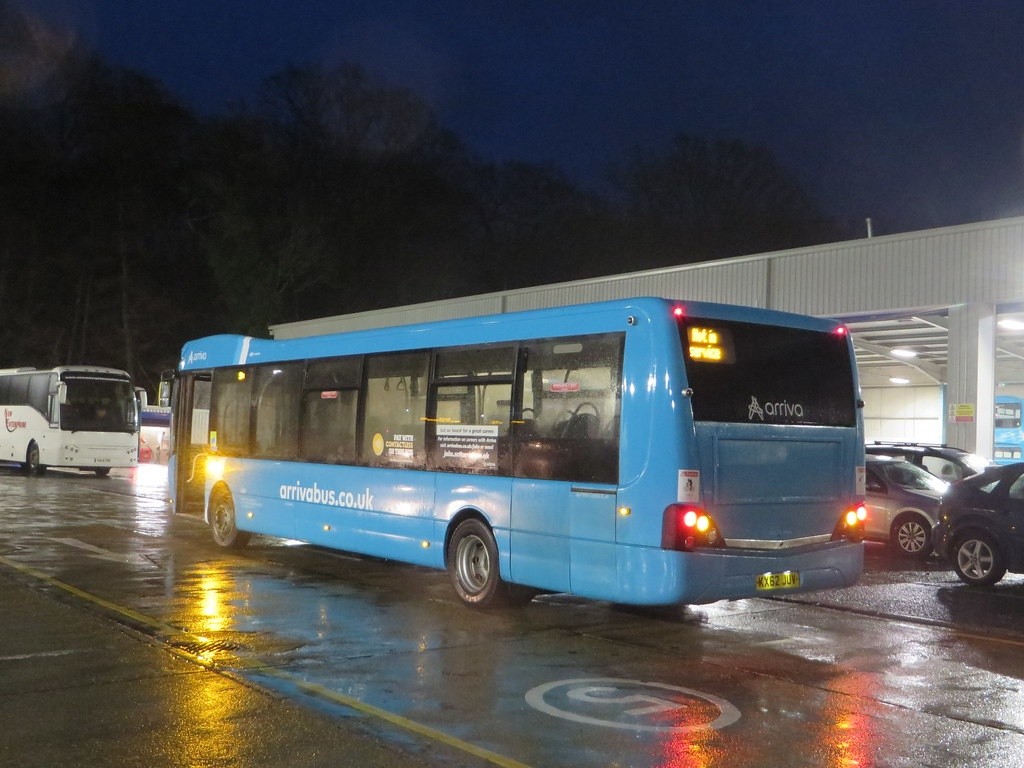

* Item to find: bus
[0,366,147,477]
[157,296,866,609]
[995,397,1024,462]
[140,407,170,458]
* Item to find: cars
[930,459,1024,588]
[860,455,948,560]
[872,441,985,481]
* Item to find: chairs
[941,465,955,484]
[521,402,620,439]
